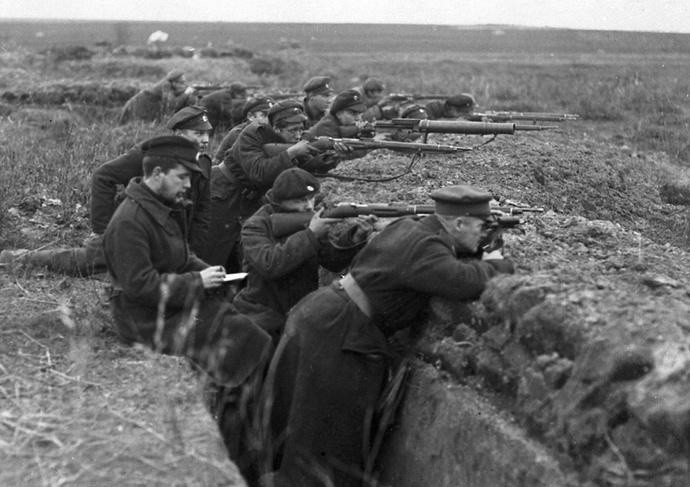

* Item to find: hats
[243,98,271,117]
[446,96,473,109]
[166,70,185,82]
[304,76,335,95]
[330,89,366,115]
[431,185,493,216]
[401,104,432,120]
[140,136,203,172]
[268,100,308,128]
[272,168,320,199]
[230,82,247,93]
[169,106,213,131]
[363,77,386,91]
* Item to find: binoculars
[478,213,521,253]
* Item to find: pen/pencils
[191,254,225,278]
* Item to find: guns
[363,115,559,139]
[270,196,544,238]
[389,92,454,102]
[260,135,473,157]
[475,111,581,122]
[190,83,265,91]
[267,92,306,99]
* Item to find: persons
[86,66,476,305]
[231,163,380,346]
[246,183,514,486]
[98,134,272,473]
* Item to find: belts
[219,160,257,200]
[339,272,379,320]
